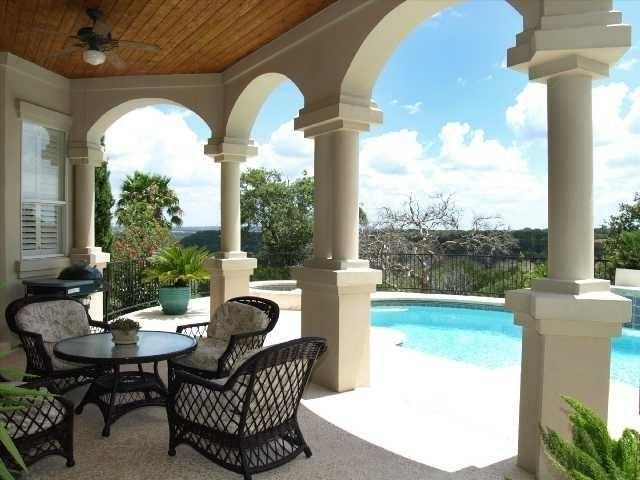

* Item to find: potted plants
[108,316,142,345]
[136,240,214,314]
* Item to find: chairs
[163,335,328,480]
[4,296,110,416]
[1,379,77,479]
[168,296,280,388]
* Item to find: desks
[21,277,97,346]
[53,331,197,439]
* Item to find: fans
[17,8,159,76]
